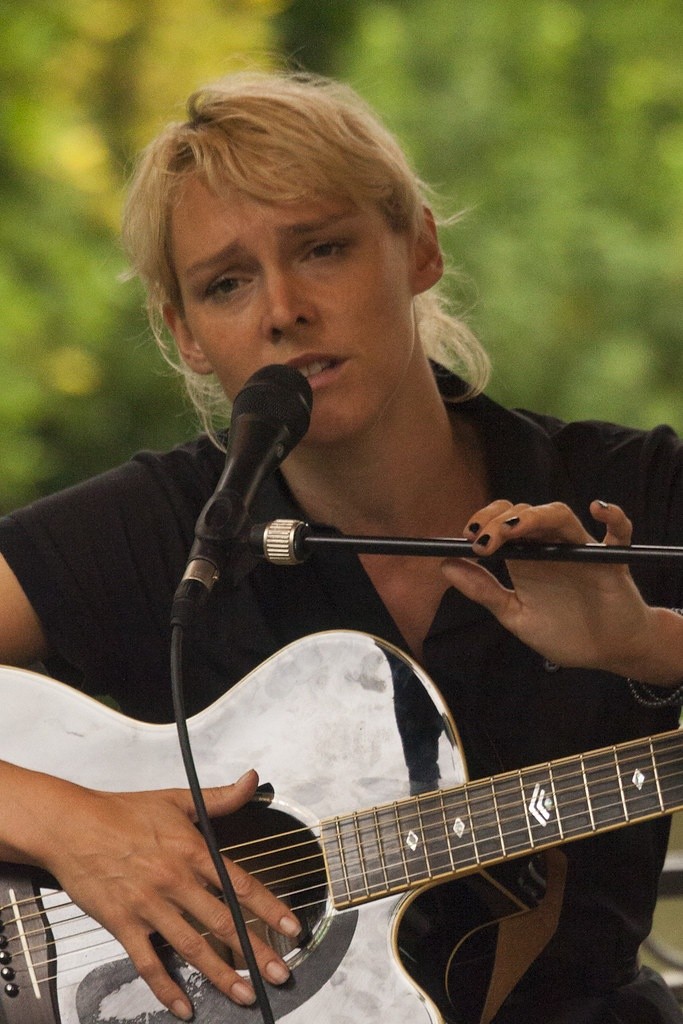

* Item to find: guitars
[0,629,683,1024]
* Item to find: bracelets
[627,607,683,710]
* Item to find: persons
[0,70,683,1024]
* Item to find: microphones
[170,363,314,631]
